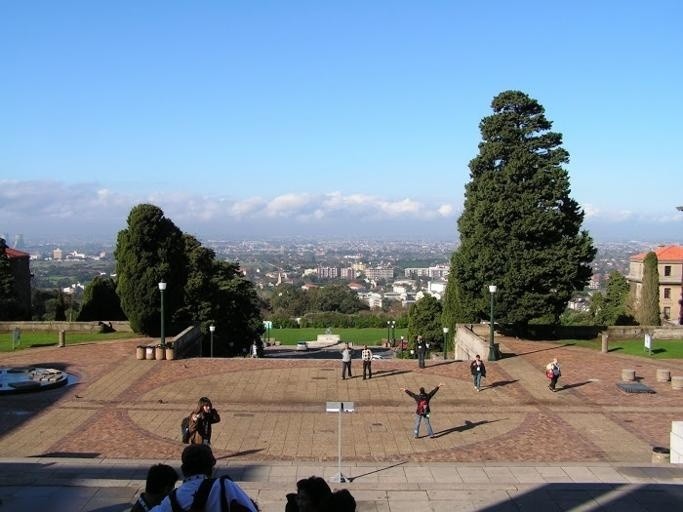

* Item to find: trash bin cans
[651,447,670,465]
[136,345,175,361]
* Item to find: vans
[296,341,308,352]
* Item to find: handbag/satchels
[471,367,475,375]
[230,499,259,512]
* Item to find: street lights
[410,349,415,359]
[209,323,216,356]
[443,327,449,360]
[387,320,396,342]
[488,282,497,360]
[158,278,167,346]
[426,343,430,359]
[401,335,405,359]
[242,347,247,358]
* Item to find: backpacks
[418,400,428,416]
[546,363,554,378]
[181,417,190,444]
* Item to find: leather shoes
[363,377,371,380]
[341,375,352,380]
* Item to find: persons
[545,358,562,393]
[399,381,446,440]
[469,355,486,392]
[339,341,373,380]
[249,339,259,358]
[181,397,221,444]
[416,336,426,368]
[129,443,356,512]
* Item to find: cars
[372,355,384,360]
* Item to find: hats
[181,443,216,467]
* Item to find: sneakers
[473,386,480,392]
[548,386,556,392]
[429,436,434,439]
[413,435,419,439]
